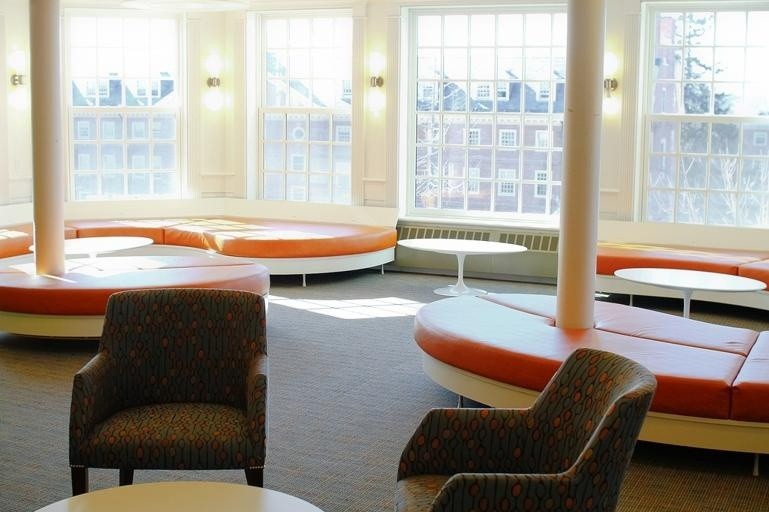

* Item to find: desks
[398,238,527,297]
[614,267,767,318]
[27,236,154,258]
[32,481,324,512]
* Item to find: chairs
[68,287,268,495]
[395,347,659,511]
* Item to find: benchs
[0,216,397,286]
[1,254,271,343]
[412,293,769,477]
[594,243,769,307]
